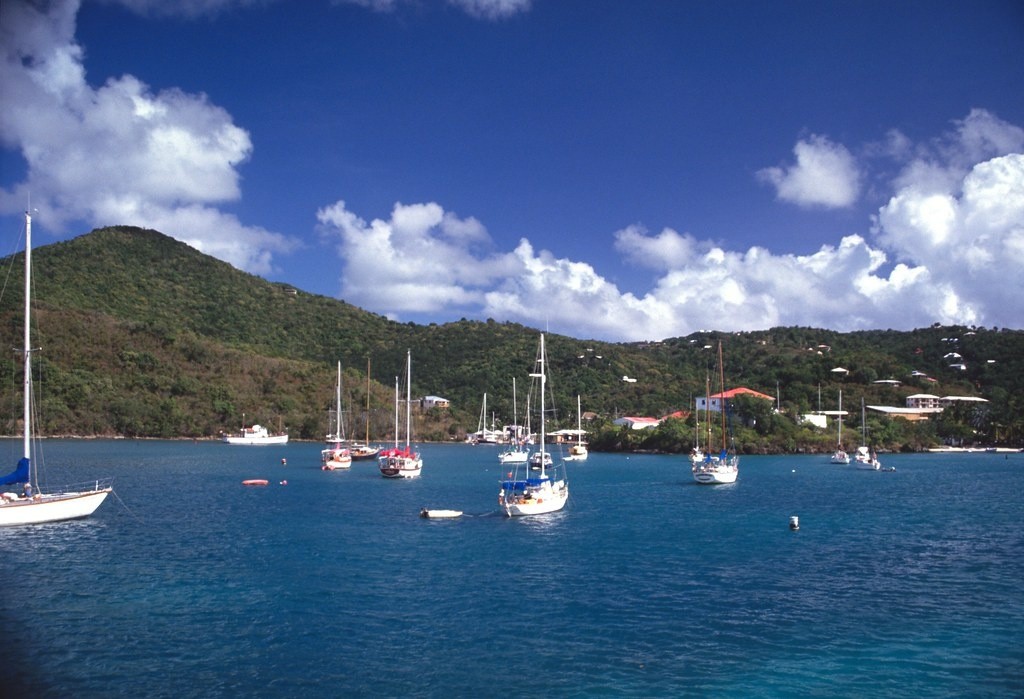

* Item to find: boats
[223,424,289,447]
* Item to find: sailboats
[497,332,570,518]
[378,348,425,479]
[0,207,117,530]
[563,394,590,463]
[831,388,853,465]
[682,339,741,485]
[471,374,555,473]
[322,345,352,473]
[349,354,383,460]
[854,396,883,470]
